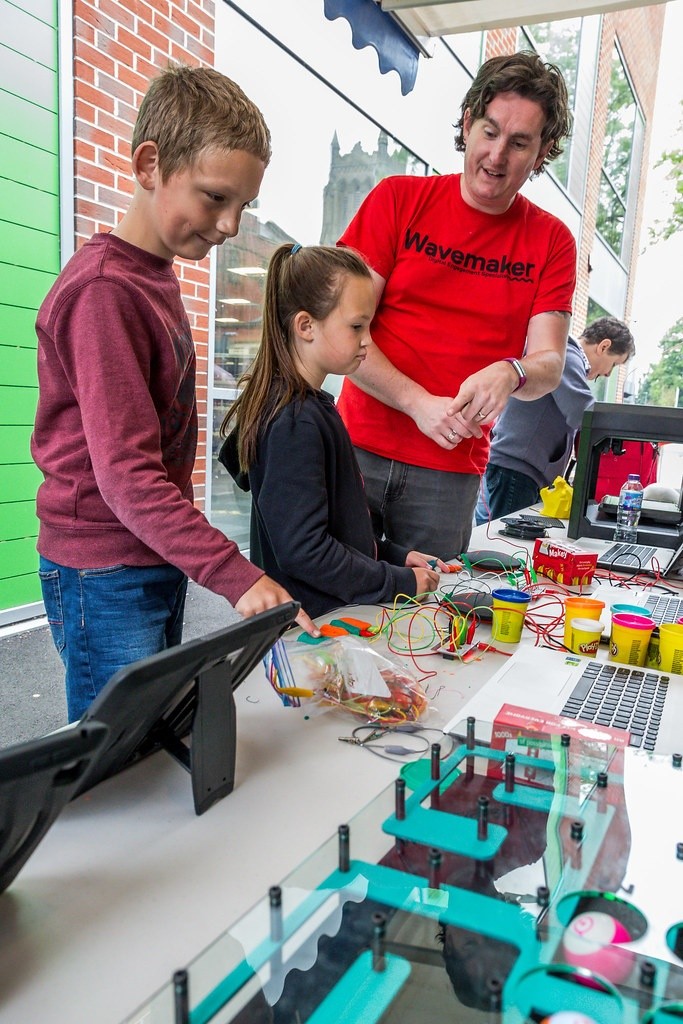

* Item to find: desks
[0,496,683,1024]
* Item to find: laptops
[443,644,683,762]
[598,475,683,523]
[569,536,683,579]
[588,584,683,644]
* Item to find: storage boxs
[488,701,631,806]
[531,538,598,585]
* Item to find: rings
[479,410,486,421]
[447,430,456,441]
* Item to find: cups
[645,627,662,669]
[658,624,683,675]
[610,604,653,618]
[492,588,531,642]
[609,613,657,668]
[570,618,605,658]
[564,597,605,649]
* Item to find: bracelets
[504,357,527,393]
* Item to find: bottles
[613,474,645,544]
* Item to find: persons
[236,242,453,627]
[26,64,324,732]
[331,55,577,562]
[485,316,637,520]
